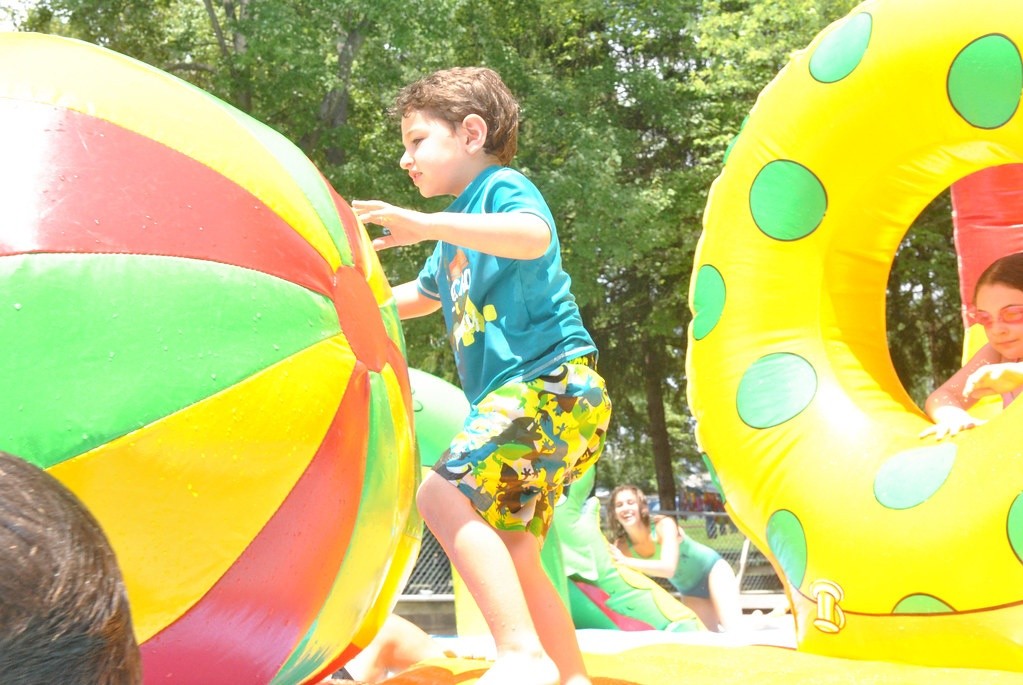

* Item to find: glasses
[974,306,1023,325]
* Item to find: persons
[332,67,612,685]
[0,449,142,685]
[919,252,1023,441]
[608,485,767,634]
